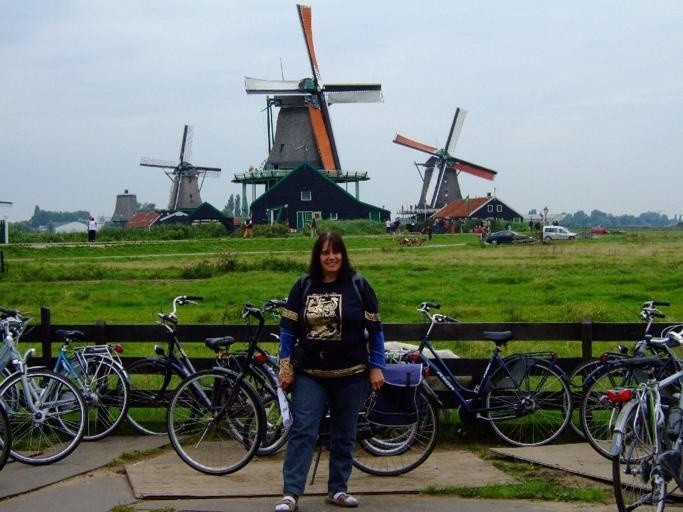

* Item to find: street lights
[2,212,9,244]
[543,206,548,225]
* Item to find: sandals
[325,491,359,508]
[274,492,298,511]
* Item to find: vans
[542,225,579,246]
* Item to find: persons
[243,216,253,239]
[89,217,96,242]
[309,218,319,238]
[529,218,559,230]
[86,216,92,242]
[385,218,433,240]
[272,231,385,512]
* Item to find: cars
[484,230,533,245]
[590,225,609,233]
[612,229,626,234]
